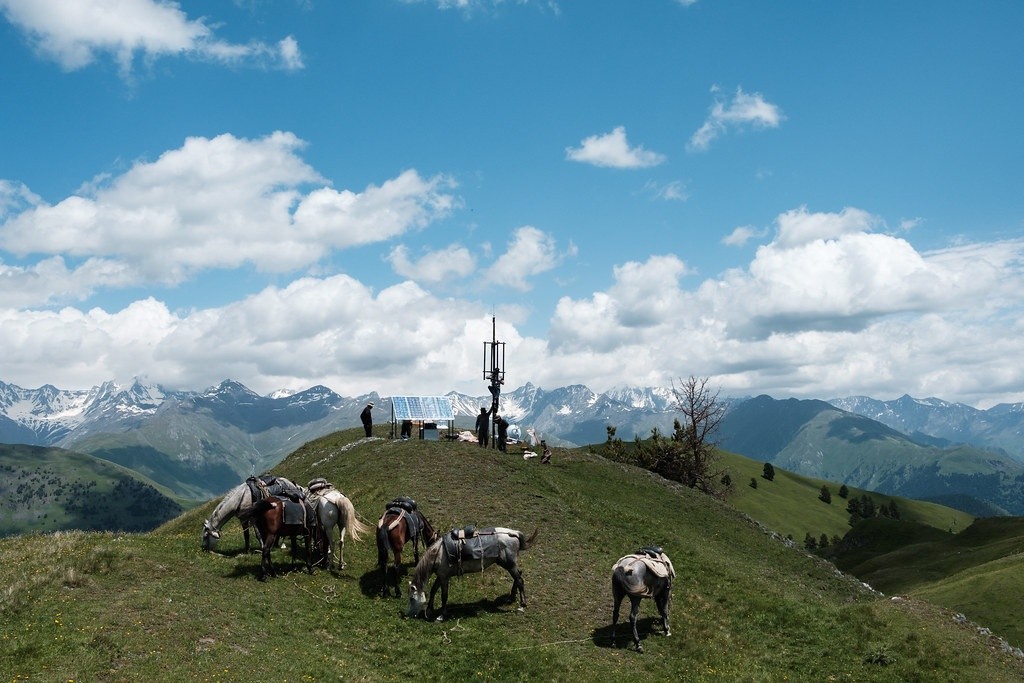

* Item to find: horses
[200,474,306,553]
[304,478,369,570]
[408,526,540,620]
[610,545,676,654]
[238,495,319,582]
[376,497,441,600]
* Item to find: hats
[494,415,501,420]
[368,402,374,408]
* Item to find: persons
[360,402,375,437]
[475,402,492,448]
[494,415,509,452]
[400,420,413,439]
